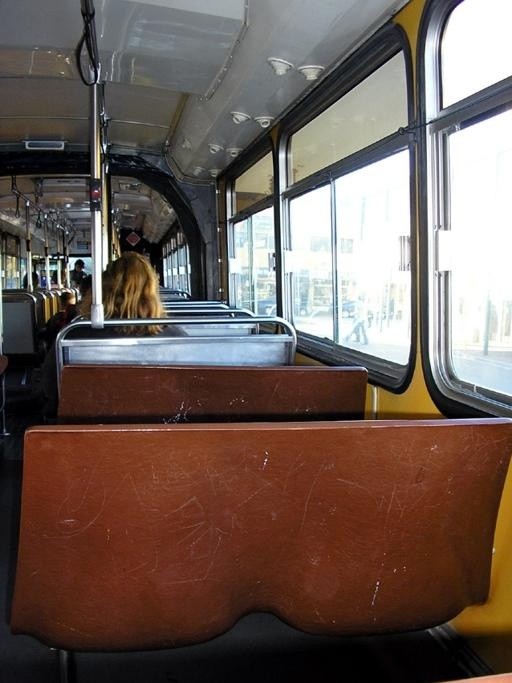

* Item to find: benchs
[3,282,512,683]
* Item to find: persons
[23,252,189,426]
[354,296,368,346]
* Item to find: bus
[232,271,363,316]
